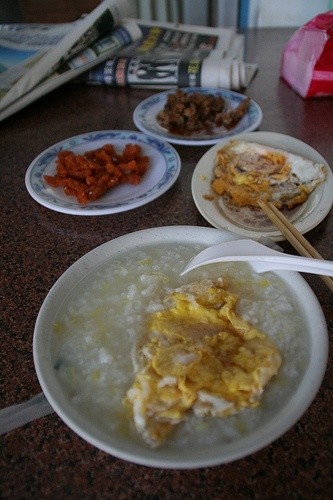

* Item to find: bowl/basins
[32,225,329,469]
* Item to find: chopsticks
[257,199,333,292]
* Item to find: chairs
[142,0,259,28]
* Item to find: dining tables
[0,27,333,500]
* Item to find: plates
[25,129,181,216]
[133,87,263,146]
[191,131,332,242]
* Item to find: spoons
[180,239,332,277]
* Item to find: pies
[211,139,327,210]
